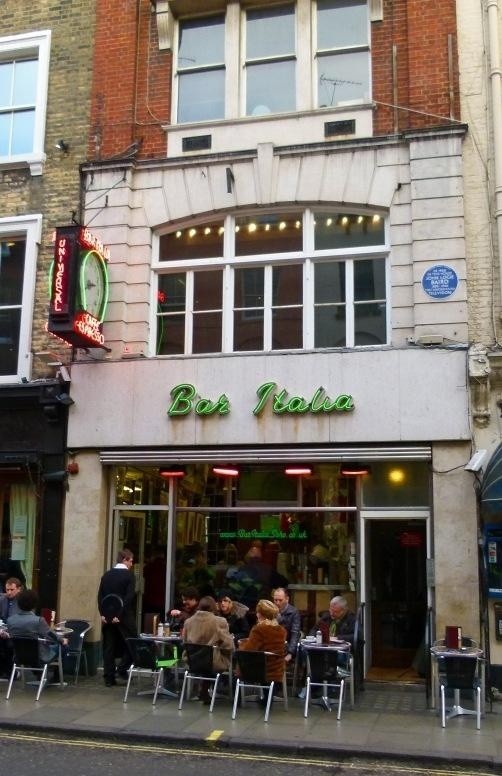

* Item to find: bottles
[50,618,67,633]
[158,623,170,638]
[316,631,322,644]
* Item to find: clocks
[41,222,115,355]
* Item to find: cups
[306,636,314,640]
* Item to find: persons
[233,598,287,708]
[270,586,301,663]
[167,585,202,624]
[215,589,252,641]
[97,548,139,687]
[180,595,241,708]
[298,596,363,700]
[5,588,69,686]
[0,577,25,621]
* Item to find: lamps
[156,462,373,480]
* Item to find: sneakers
[105,663,134,686]
[35,674,71,688]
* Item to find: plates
[303,640,316,643]
